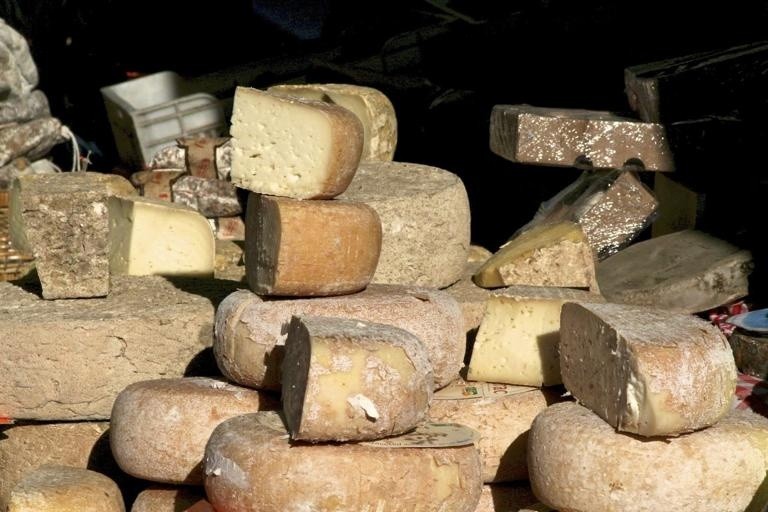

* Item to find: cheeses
[0,18,768,512]
